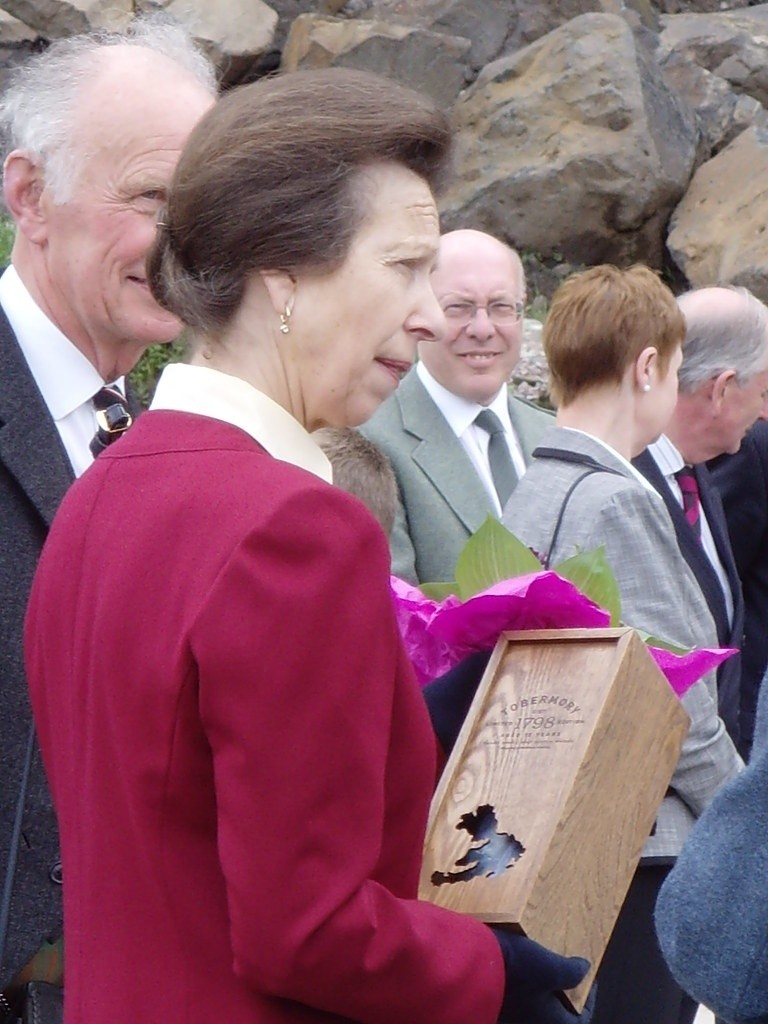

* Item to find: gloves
[490,928,598,1024]
[422,653,491,753]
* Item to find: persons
[23,65,599,1024]
[307,230,768,1024]
[0,28,216,1024]
[654,671,768,1023]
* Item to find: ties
[674,465,701,541]
[88,384,133,462]
[472,407,519,514]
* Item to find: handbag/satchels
[0,981,64,1024]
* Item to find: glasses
[441,301,527,327]
